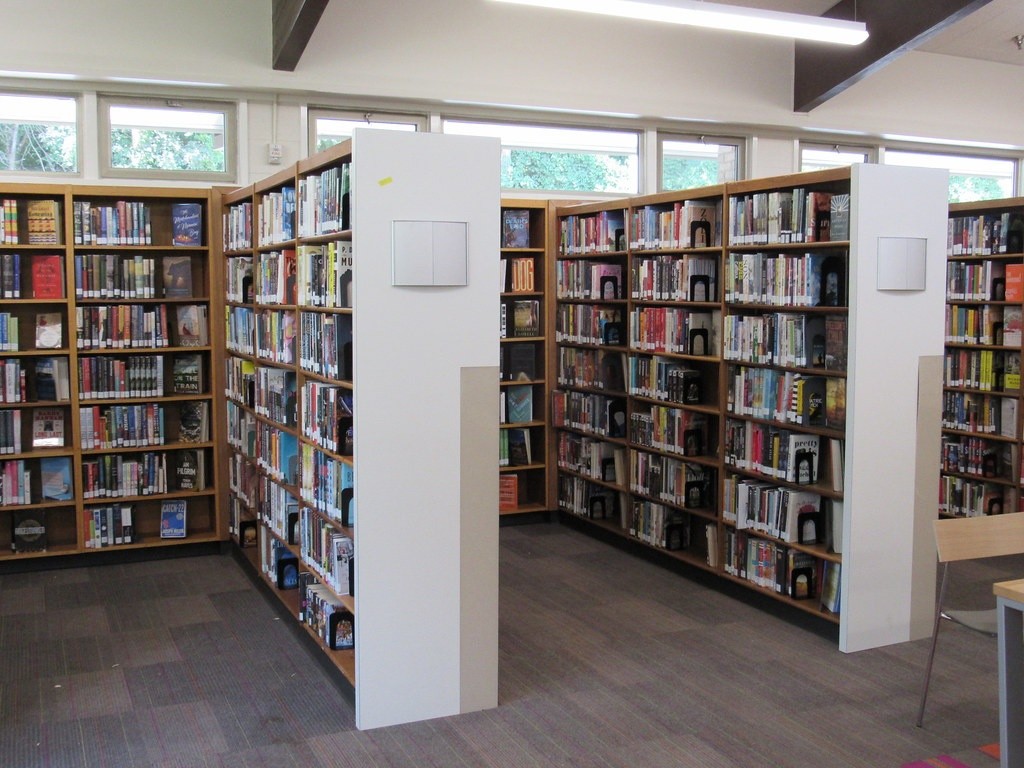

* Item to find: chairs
[916,510,1024,730]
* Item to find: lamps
[486,0,869,48]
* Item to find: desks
[991,577,1024,768]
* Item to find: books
[939,214,1024,518]
[551,188,849,616]
[500,209,538,510]
[0,199,210,552]
[223,162,353,650]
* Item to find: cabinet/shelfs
[0,127,1024,731]
